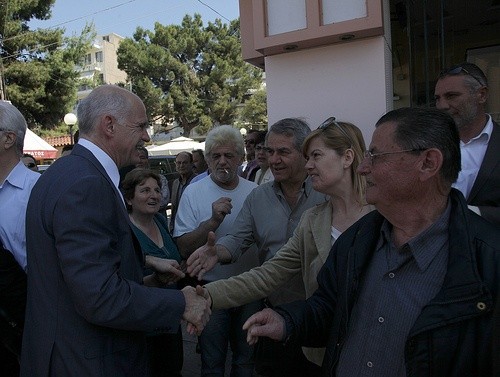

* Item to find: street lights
[64,112,77,145]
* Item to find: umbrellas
[147,136,201,156]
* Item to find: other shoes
[195,341,201,353]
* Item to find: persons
[171,124,260,377]
[186,117,377,377]
[18,85,211,377]
[241,129,275,184]
[22,154,37,171]
[187,117,330,377]
[121,168,180,277]
[435,64,500,226]
[242,107,500,377]
[0,100,42,274]
[171,149,210,232]
[132,147,170,217]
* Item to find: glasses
[318,117,352,148]
[254,146,266,153]
[364,148,426,167]
[438,66,485,87]
[244,140,257,145]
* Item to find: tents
[23,128,59,160]
